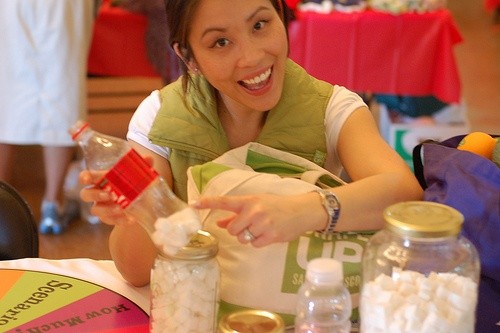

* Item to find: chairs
[0,179,39,262]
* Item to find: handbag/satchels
[412,134,500,278]
[186,142,379,333]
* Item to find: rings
[245,228,256,241]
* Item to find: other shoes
[39,197,79,235]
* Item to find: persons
[80,0,423,287]
[0,0,96,234]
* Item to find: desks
[285,0,463,104]
[0,260,151,333]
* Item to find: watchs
[313,189,340,234]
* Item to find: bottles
[294,257,353,333]
[148,229,222,333]
[358,200,480,333]
[67,119,202,257]
